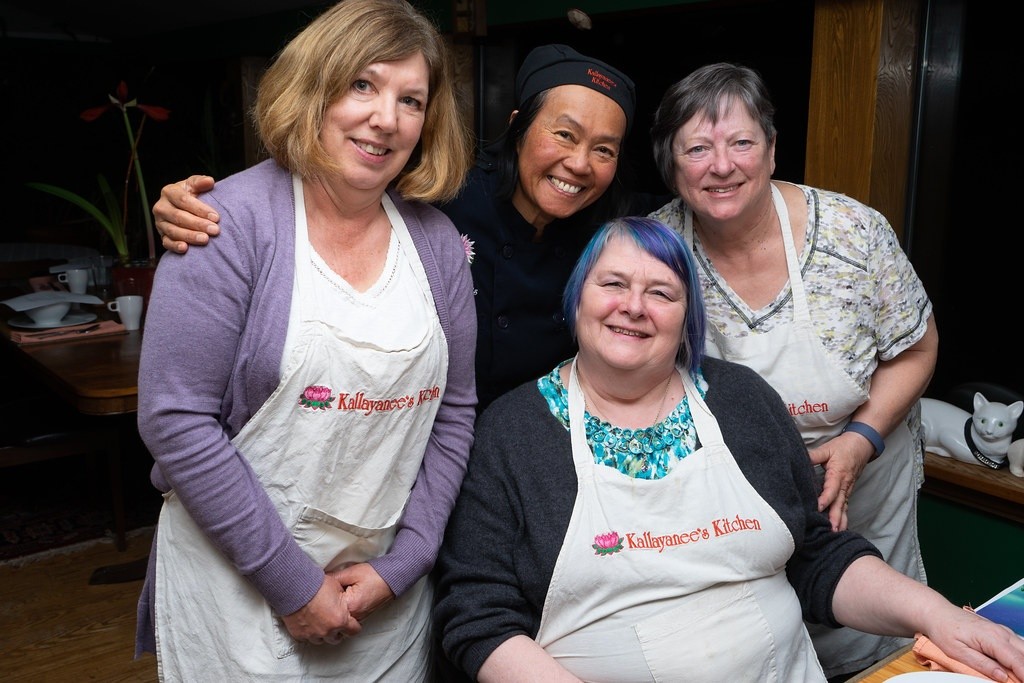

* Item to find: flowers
[79,80,170,268]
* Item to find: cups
[59,269,89,296]
[106,294,143,331]
[92,256,114,288]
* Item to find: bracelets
[840,421,886,463]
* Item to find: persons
[428,216,1023,682]
[641,62,941,683]
[150,45,645,429]
[133,1,478,683]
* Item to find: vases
[110,259,157,325]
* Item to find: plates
[9,313,98,330]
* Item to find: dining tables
[1,300,149,552]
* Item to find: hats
[515,42,638,130]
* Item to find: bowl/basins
[24,300,72,325]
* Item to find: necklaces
[572,364,672,473]
